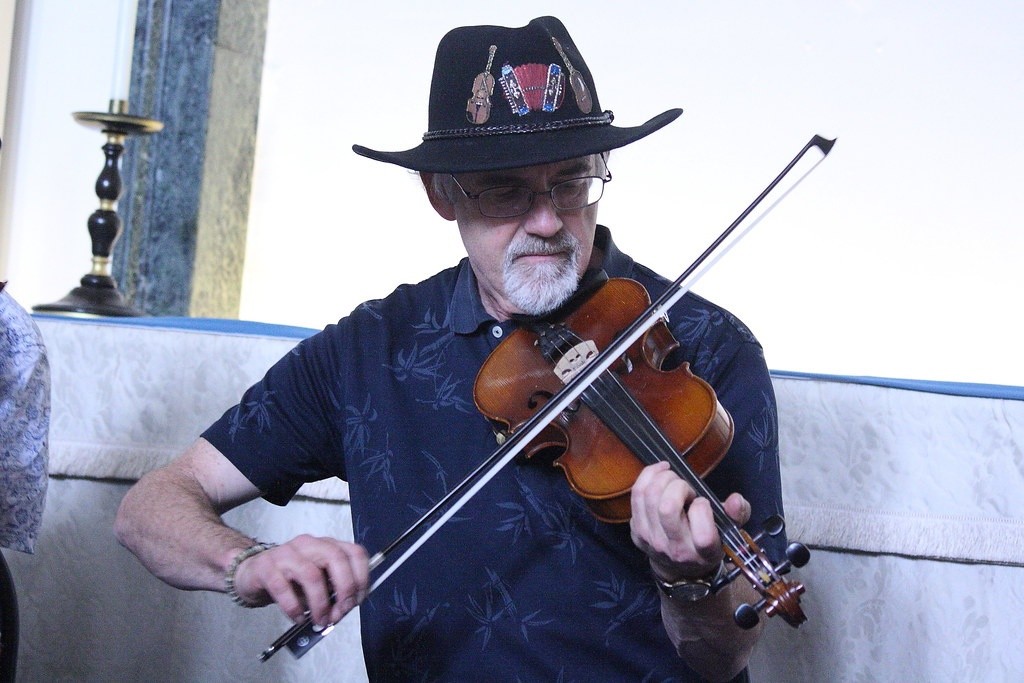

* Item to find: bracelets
[224,543,273,608]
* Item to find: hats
[351,18,684,174]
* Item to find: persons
[115,17,794,683]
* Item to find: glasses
[450,153,612,219]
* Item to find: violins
[475,273,815,631]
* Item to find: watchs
[649,559,729,603]
